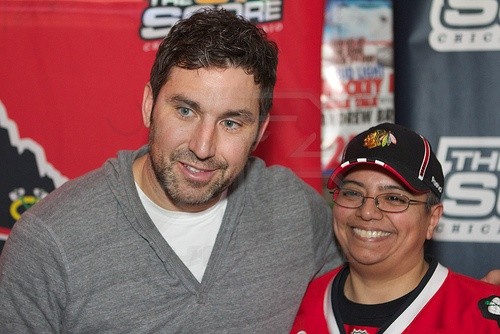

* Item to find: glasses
[328,188,428,214]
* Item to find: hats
[327,123,444,203]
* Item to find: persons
[290,121,500,334]
[1,6,345,333]
